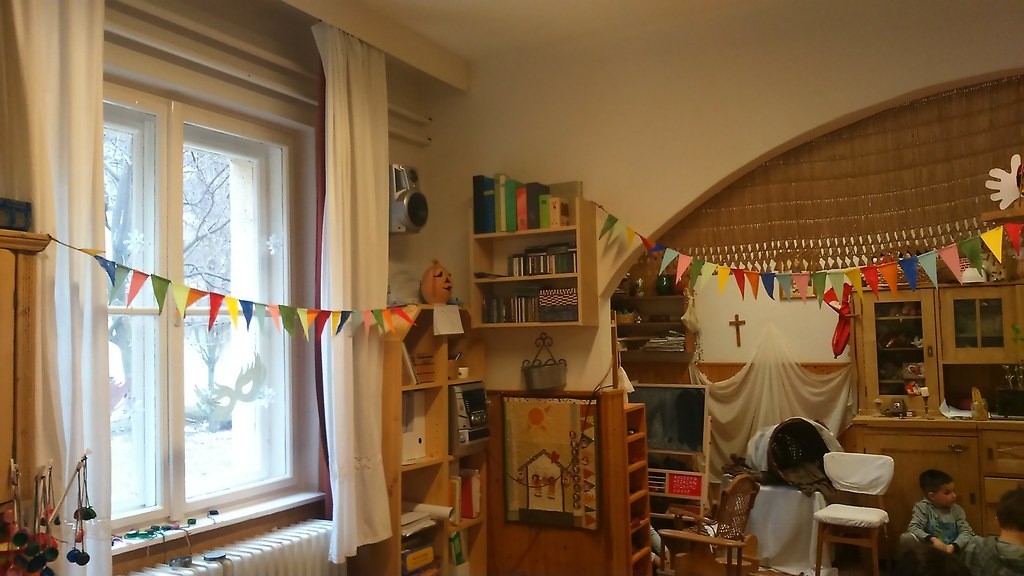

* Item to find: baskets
[767,418,832,487]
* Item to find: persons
[649,524,676,576]
[894,469,976,576]
[961,486,1024,576]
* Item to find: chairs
[669,473,761,558]
[815,452,895,576]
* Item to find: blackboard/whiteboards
[624,383,710,456]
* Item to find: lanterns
[421,259,454,305]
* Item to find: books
[394,174,686,576]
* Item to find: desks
[656,529,746,576]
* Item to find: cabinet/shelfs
[348,306,487,576]
[486,389,652,576]
[618,275,697,353]
[850,280,1024,539]
[469,174,600,330]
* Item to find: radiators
[128,519,346,576]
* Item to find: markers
[454,351,462,360]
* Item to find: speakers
[388,164,429,234]
